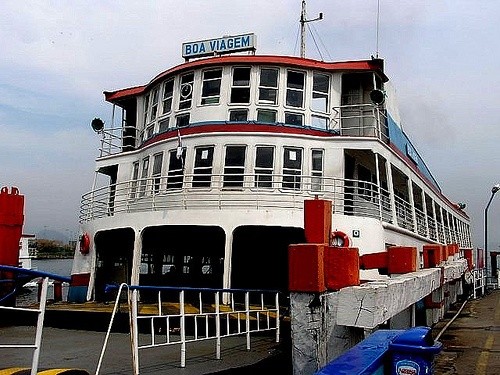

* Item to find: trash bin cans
[387,326,443,375]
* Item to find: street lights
[484,182,500,296]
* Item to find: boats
[0,0,478,375]
